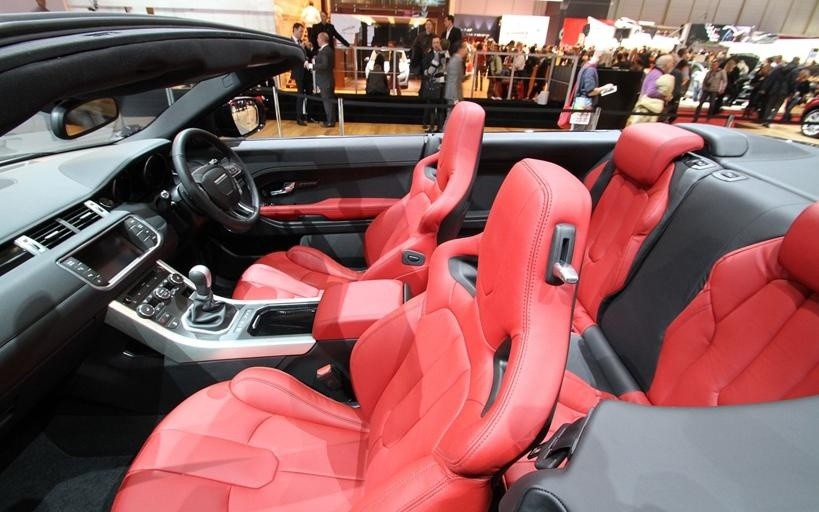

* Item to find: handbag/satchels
[422,81,441,99]
[557,105,573,129]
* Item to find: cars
[801,94,819,138]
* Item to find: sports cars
[0,7,819,512]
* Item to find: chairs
[106,158,592,512]
[449,121,708,329]
[503,200,819,488]
[232,99,489,304]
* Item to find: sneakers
[321,121,335,127]
[298,118,306,126]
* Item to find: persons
[304,30,338,128]
[365,65,389,96]
[310,10,353,59]
[387,40,402,96]
[371,44,385,71]
[290,23,318,127]
[411,13,818,133]
